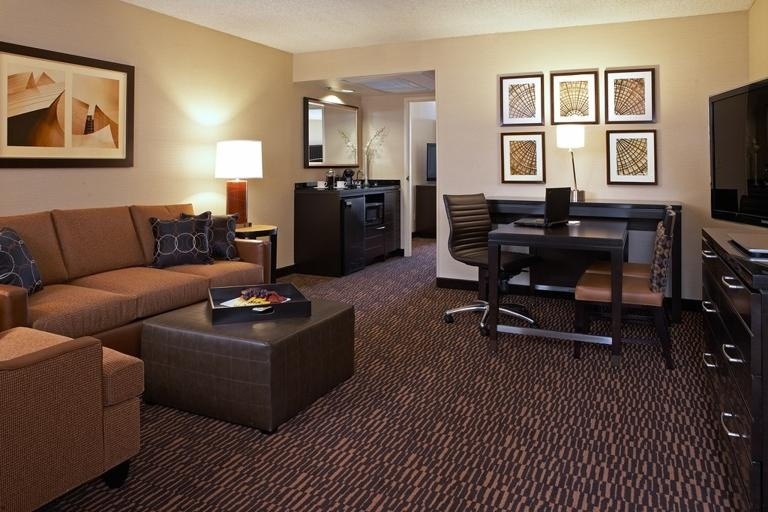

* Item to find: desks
[139,298,357,431]
[486,195,683,324]
[486,217,629,355]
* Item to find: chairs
[572,201,677,370]
[439,191,539,337]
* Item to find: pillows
[147,210,214,268]
[180,212,242,261]
[0,224,45,297]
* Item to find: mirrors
[302,95,361,169]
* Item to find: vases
[363,154,371,188]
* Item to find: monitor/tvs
[427,143,436,180]
[709,78,768,255]
[310,145,322,162]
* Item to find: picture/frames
[0,40,138,169]
[500,131,548,186]
[605,129,658,186]
[549,69,599,127]
[499,72,545,127]
[604,67,655,127]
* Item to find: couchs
[0,202,273,512]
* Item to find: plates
[333,186,348,190]
[313,186,328,191]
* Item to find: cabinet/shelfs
[698,222,766,512]
[293,178,401,277]
[414,184,436,239]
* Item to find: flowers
[363,125,387,163]
[336,125,357,164]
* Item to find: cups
[317,181,327,187]
[337,181,347,187]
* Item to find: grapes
[241,287,274,300]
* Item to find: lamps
[212,139,264,229]
[555,124,586,203]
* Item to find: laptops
[514,188,571,228]
